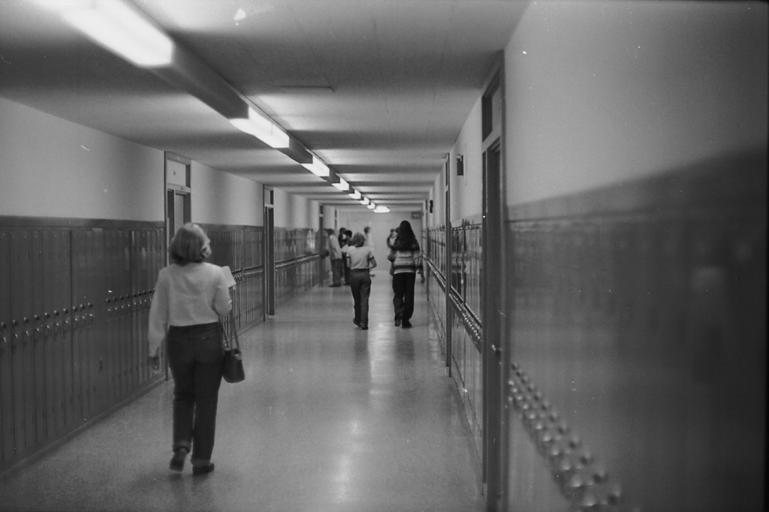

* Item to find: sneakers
[194,462,216,476]
[396,311,412,328]
[168,447,188,471]
[354,319,368,329]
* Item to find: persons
[323,223,399,289]
[345,232,377,330]
[146,219,235,478]
[387,219,426,329]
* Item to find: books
[222,265,237,293]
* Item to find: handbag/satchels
[222,348,244,382]
